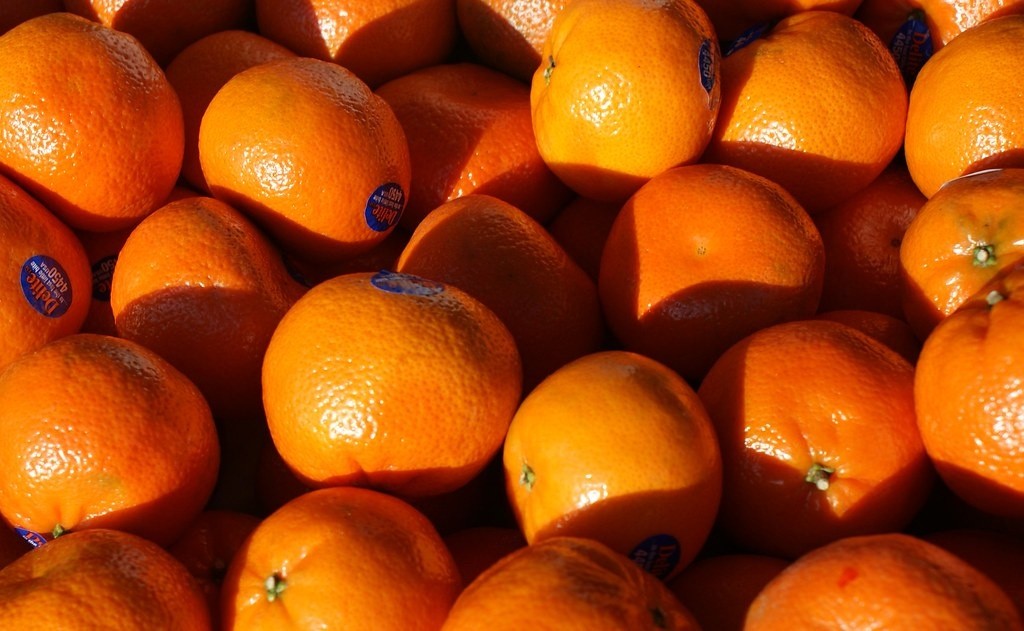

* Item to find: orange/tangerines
[0,0,1024,631]
